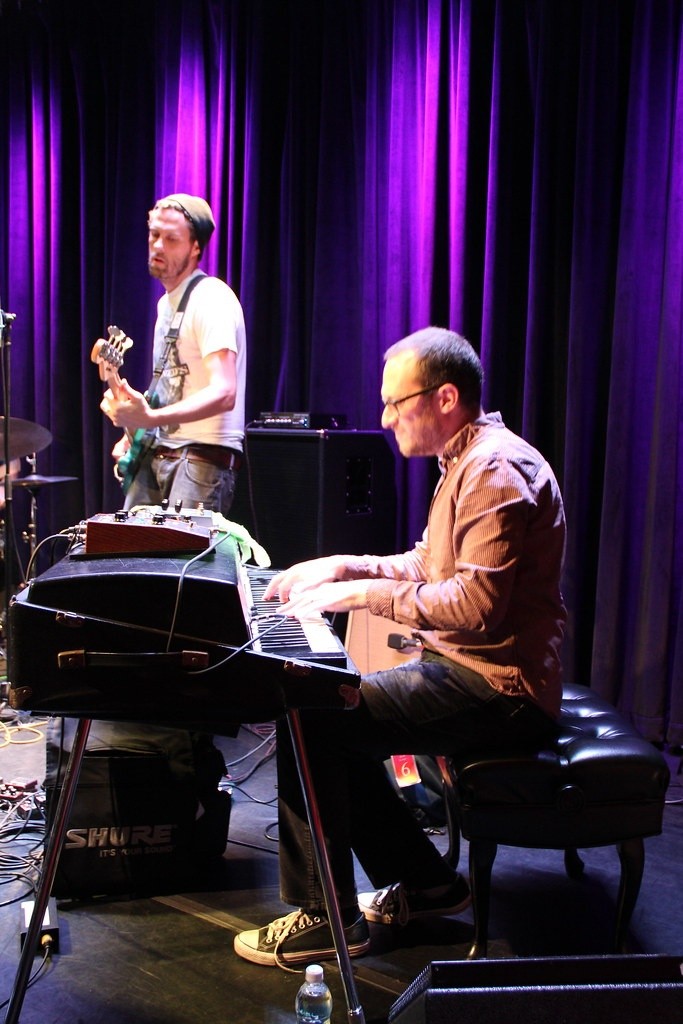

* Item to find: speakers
[243,428,398,572]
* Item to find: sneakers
[234,906,374,974]
[356,869,475,924]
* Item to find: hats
[161,193,216,262]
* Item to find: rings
[104,410,109,413]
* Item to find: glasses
[385,383,440,418]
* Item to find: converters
[20,896,59,954]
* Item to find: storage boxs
[45,716,233,898]
[243,422,399,569]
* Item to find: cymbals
[0,411,53,468]
[1,474,76,490]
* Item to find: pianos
[4,502,361,730]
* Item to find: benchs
[413,684,670,960]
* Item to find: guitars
[90,321,161,513]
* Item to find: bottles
[296,965,333,1024]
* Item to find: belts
[152,444,244,472]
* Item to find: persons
[235,326,567,966]
[99,195,248,514]
